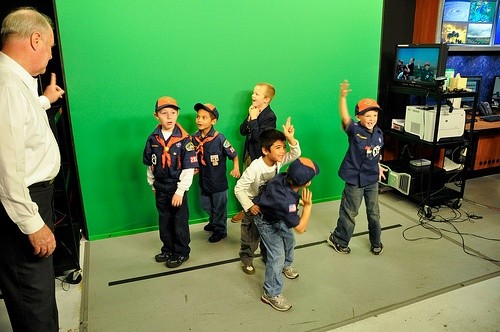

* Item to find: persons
[327,80,388,256]
[252,156,320,311]
[191,103,240,243]
[0,7,65,332]
[418,61,434,82]
[143,97,199,268]
[396,57,415,80]
[234,116,301,275]
[231,83,277,223]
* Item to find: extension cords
[424,205,432,217]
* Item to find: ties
[155,122,188,169]
[195,131,218,166]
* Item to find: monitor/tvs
[393,43,449,89]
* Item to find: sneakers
[166,254,189,268]
[327,234,351,253]
[209,233,227,242]
[282,265,299,278]
[155,251,172,262]
[231,212,244,223]
[371,245,383,255]
[242,263,255,274]
[204,224,213,231]
[261,290,292,312]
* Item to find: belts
[28,180,54,188]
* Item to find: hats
[155,96,180,111]
[355,98,382,116]
[399,60,403,64]
[194,103,219,120]
[404,71,409,75]
[288,157,319,186]
[425,62,430,66]
